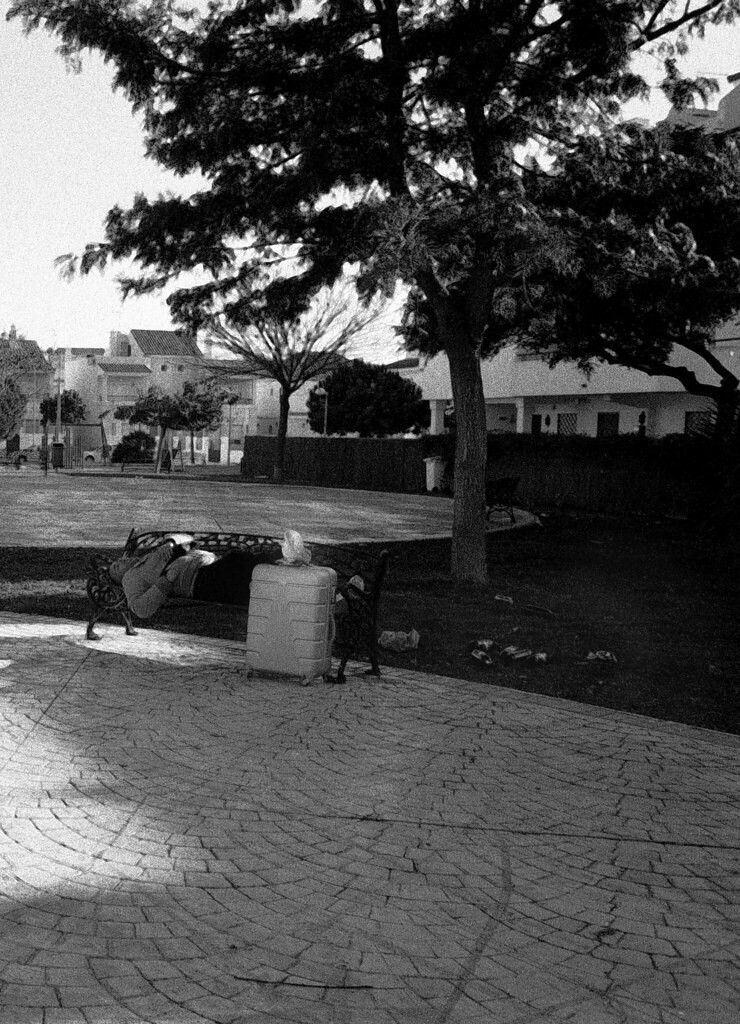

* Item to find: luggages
[243,558,336,686]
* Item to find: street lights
[316,388,329,437]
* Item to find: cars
[83,446,122,462]
[11,444,69,466]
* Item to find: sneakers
[281,530,313,563]
[339,574,364,614]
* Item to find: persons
[105,530,377,618]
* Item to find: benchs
[485,475,517,527]
[86,524,387,681]
